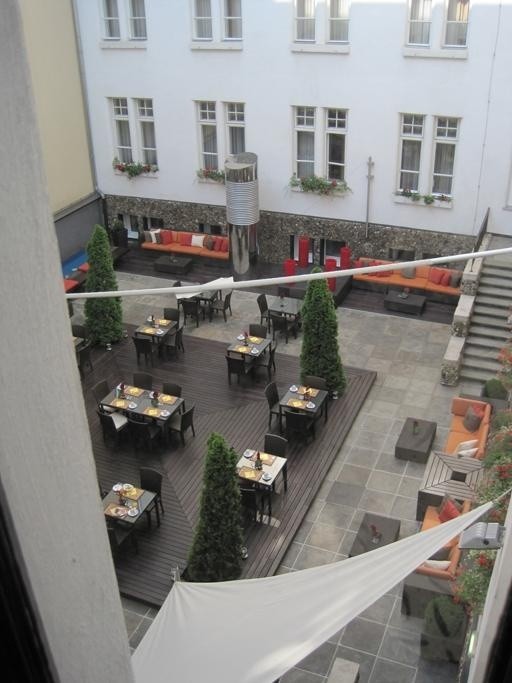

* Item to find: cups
[131,401,135,407]
[264,472,270,479]
[253,347,257,352]
[292,384,296,390]
[158,329,161,333]
[308,402,312,407]
[245,449,251,455]
[162,409,167,415]
[148,316,153,320]
[240,334,244,339]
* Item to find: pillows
[143,229,229,254]
[453,400,485,459]
[423,492,464,571]
[354,257,462,290]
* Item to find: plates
[122,484,134,491]
[290,387,298,392]
[157,331,163,335]
[262,473,273,481]
[244,450,254,458]
[147,318,155,322]
[307,404,316,408]
[160,412,170,417]
[113,484,122,492]
[237,336,245,341]
[251,350,259,354]
[129,404,137,409]
[128,509,139,516]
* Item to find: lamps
[458,522,507,551]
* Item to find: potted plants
[111,217,129,248]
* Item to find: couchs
[414,497,473,579]
[139,227,232,268]
[351,255,463,303]
[446,394,493,461]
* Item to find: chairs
[223,323,280,387]
[229,431,292,526]
[255,285,309,345]
[170,281,233,328]
[90,371,198,456]
[129,306,186,366]
[89,464,170,553]
[263,374,330,444]
